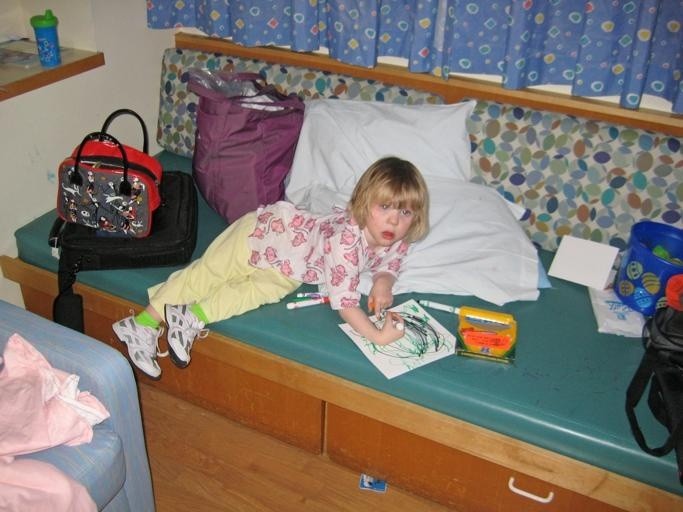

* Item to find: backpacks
[623,307,682,483]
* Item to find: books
[0,48,42,69]
[0,36,66,56]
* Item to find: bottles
[30,8,63,68]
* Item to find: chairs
[2,298,157,510]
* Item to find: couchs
[1,29,682,510]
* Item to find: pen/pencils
[456,347,516,364]
[286,292,329,310]
[417,300,460,315]
[380,308,403,331]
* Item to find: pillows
[300,178,544,309]
[286,93,477,197]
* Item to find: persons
[109,154,430,379]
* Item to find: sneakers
[163,303,209,367]
[111,309,169,382]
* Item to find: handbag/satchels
[55,109,164,237]
[188,74,306,226]
[48,170,200,336]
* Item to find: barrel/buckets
[613,221,683,317]
